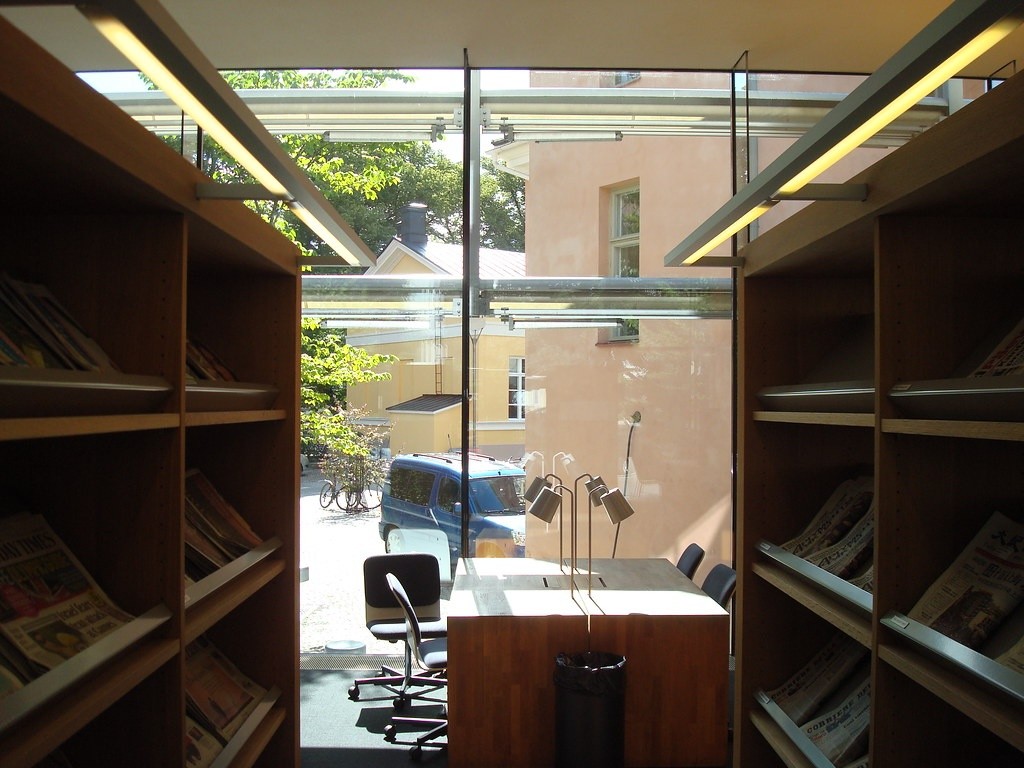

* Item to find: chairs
[383,572,447,761]
[676,543,706,580]
[701,563,737,609]
[348,553,448,713]
[388,527,454,587]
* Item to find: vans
[379,450,525,577]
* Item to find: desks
[565,558,731,768]
[446,558,588,768]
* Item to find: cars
[300,454,309,471]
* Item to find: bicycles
[317,474,384,510]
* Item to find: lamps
[520,450,581,532]
[663,0,1024,269]
[589,484,636,597]
[528,484,575,598]
[574,473,608,568]
[524,474,563,572]
[0,0,378,269]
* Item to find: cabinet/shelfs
[733,71,1024,768]
[0,16,304,768]
